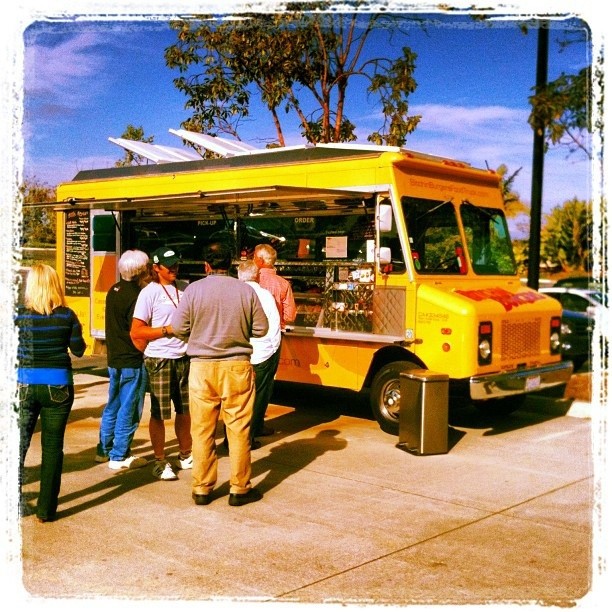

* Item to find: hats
[152,246,184,267]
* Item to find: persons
[131,247,193,479]
[169,242,270,507]
[248,244,298,437]
[95,247,151,472]
[15,262,89,523]
[225,257,284,452]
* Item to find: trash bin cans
[396,369,449,456]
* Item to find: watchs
[162,326,168,336]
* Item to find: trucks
[53,128,574,436]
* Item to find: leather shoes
[228,488,262,506]
[192,494,210,504]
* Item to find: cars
[539,286,606,318]
[561,310,595,372]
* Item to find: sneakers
[173,456,193,469]
[110,457,148,469]
[152,460,176,479]
[250,439,261,449]
[255,427,274,437]
[93,455,109,462]
